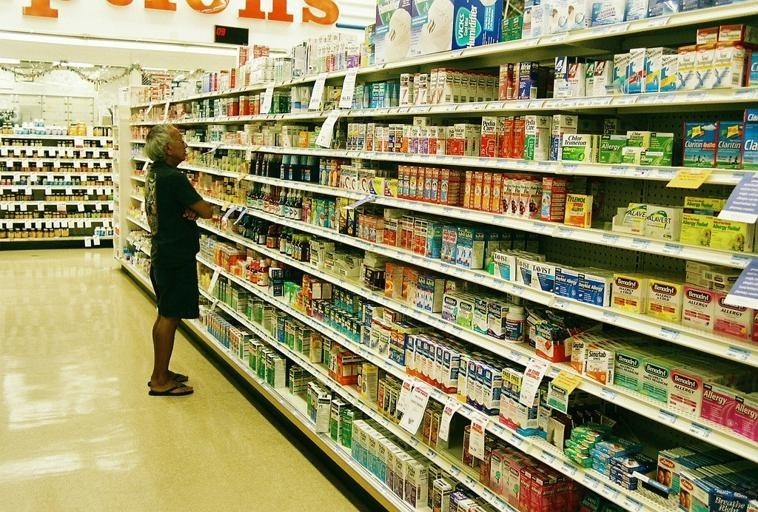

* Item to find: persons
[141,121,213,395]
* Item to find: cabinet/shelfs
[117,2,757,511]
[0,120,114,248]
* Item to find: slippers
[148,374,193,397]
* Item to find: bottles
[246,181,302,221]
[241,150,338,189]
[242,211,311,263]
[239,252,286,288]
[506,305,527,343]
[0,122,112,238]
